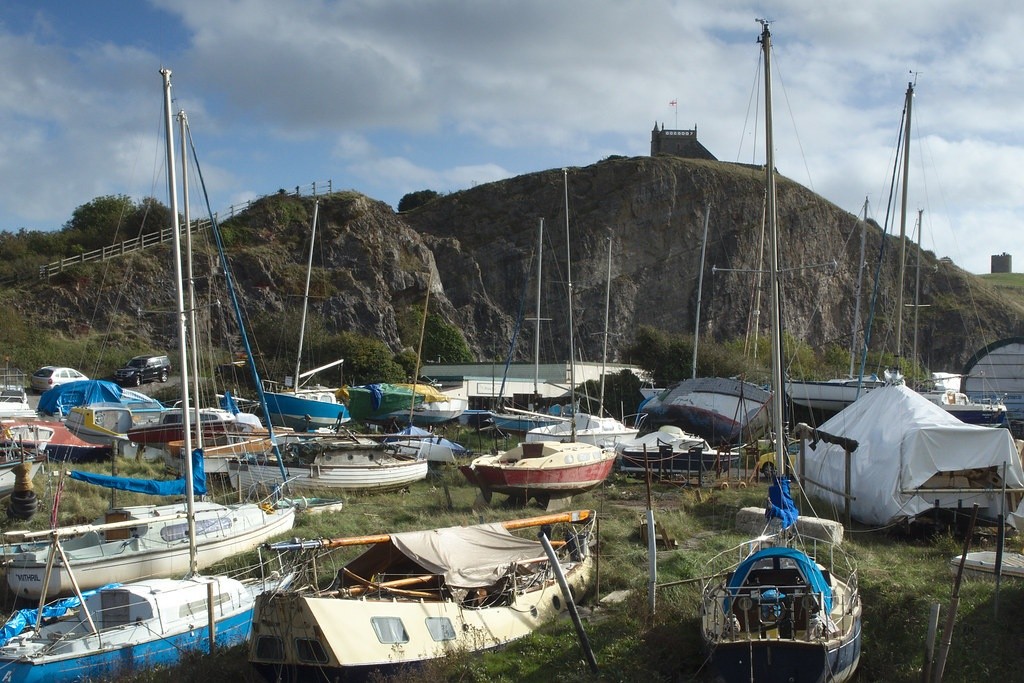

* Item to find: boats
[246,507,597,670]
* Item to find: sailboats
[0,62,1009,683]
[785,70,1024,533]
[695,19,863,683]
